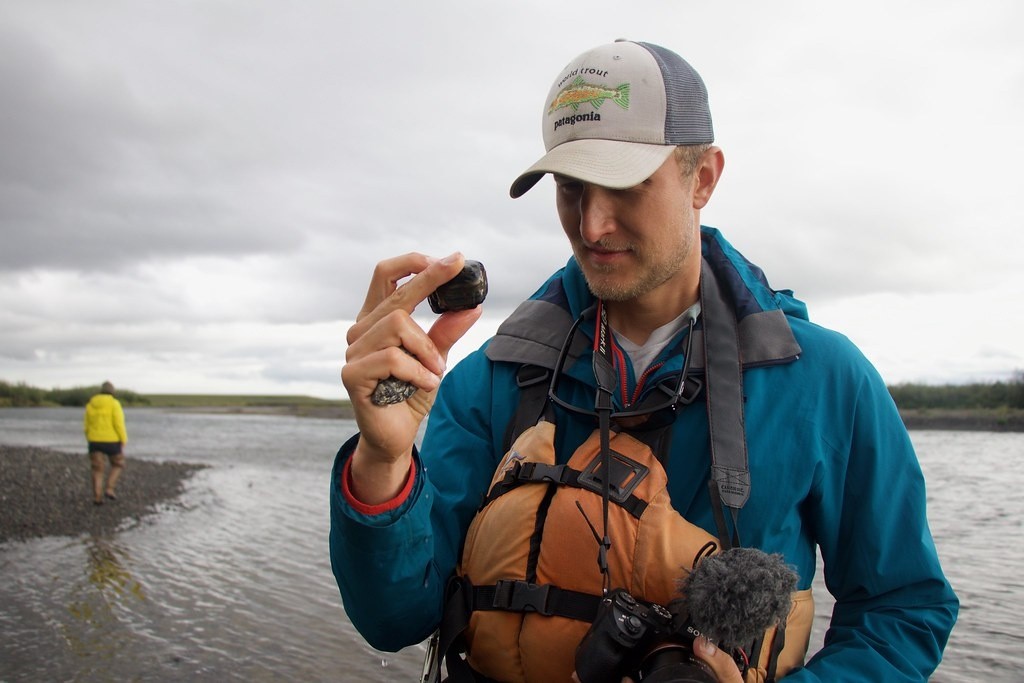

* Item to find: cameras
[574,587,746,683]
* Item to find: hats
[510,39,715,198]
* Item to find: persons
[84,381,128,505]
[329,39,961,683]
